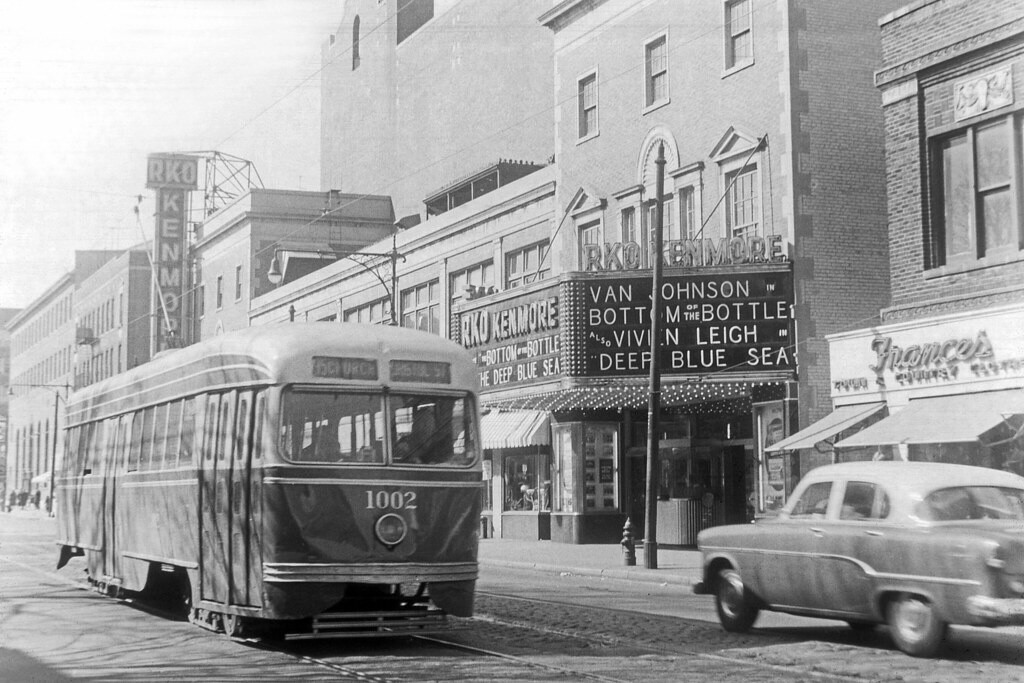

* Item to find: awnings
[763,401,886,454]
[833,387,1024,453]
[453,412,551,454]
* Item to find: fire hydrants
[621,519,636,565]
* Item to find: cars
[693,459,1024,657]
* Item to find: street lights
[6,384,69,519]
[266,247,401,326]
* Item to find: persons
[45,495,50,510]
[394,406,467,465]
[302,425,366,462]
[8,487,41,512]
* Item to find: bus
[53,322,487,654]
